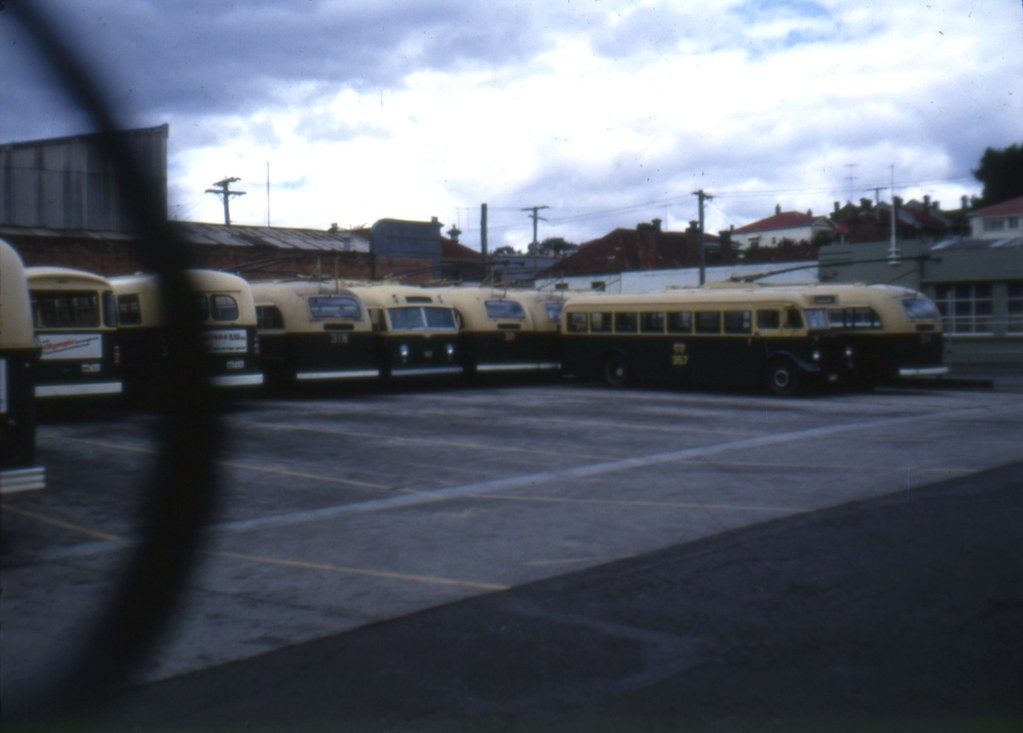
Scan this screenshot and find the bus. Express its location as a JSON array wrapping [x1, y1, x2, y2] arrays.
[[247, 278, 379, 393], [106, 268, 266, 399], [23, 265, 123, 401], [340, 280, 466, 381], [423, 283, 566, 380], [557, 279, 951, 399], [0, 239, 46, 494]]
